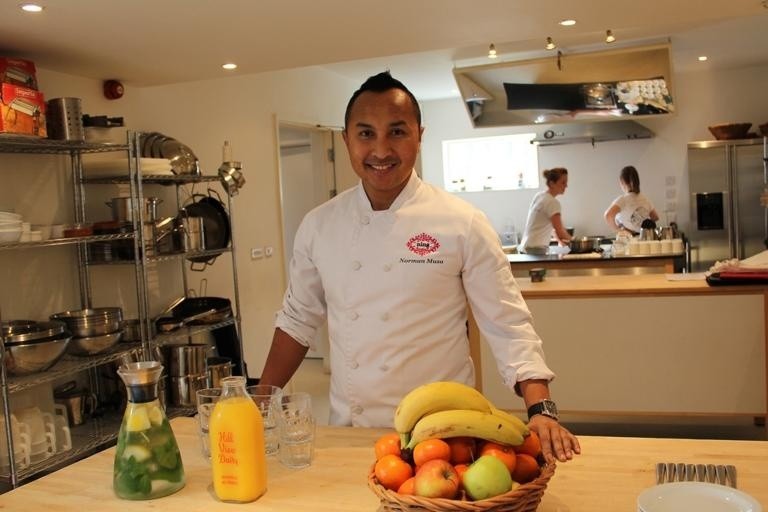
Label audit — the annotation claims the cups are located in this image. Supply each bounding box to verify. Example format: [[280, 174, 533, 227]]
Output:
[[610, 230, 628, 257], [528, 267, 546, 282], [188, 381, 314, 469], [628, 240, 682, 255]]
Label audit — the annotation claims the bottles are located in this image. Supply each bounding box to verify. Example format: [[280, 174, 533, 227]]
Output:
[[110, 356, 268, 503]]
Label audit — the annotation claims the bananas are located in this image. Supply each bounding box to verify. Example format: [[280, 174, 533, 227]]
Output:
[[392, 380, 532, 464]]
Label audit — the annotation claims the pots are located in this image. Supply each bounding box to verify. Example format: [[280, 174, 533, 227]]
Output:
[[90, 188, 234, 265], [168, 344, 237, 410], [119, 278, 235, 346]]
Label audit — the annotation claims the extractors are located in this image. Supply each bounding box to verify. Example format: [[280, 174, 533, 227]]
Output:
[[529, 118, 654, 146]]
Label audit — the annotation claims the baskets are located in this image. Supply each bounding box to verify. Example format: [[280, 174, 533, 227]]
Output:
[[368, 460, 556, 512]]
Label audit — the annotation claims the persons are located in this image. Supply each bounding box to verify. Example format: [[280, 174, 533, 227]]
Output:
[[602, 164, 660, 239], [517, 168, 573, 255], [247, 66, 584, 465]]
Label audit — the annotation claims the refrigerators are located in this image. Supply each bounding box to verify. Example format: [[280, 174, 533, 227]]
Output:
[[677, 136, 768, 272]]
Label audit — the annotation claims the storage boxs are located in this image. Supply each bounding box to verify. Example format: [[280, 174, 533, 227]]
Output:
[[0, 58, 48, 135]]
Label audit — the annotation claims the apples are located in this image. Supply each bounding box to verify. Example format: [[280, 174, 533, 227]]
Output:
[[373, 430, 543, 502]]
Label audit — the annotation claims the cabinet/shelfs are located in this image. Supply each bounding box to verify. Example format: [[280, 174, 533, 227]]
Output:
[[686, 137, 767, 275]]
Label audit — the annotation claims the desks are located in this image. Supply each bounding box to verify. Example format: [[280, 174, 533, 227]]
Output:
[[0, 415, 767, 512]]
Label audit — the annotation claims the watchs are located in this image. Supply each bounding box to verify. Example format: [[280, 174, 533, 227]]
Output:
[[527, 397, 560, 422]]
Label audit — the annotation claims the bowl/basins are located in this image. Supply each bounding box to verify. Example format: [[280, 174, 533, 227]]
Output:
[[0, 211, 67, 247], [707, 121, 753, 141], [568, 237, 601, 252], [1, 304, 126, 375], [134, 132, 201, 178]]
